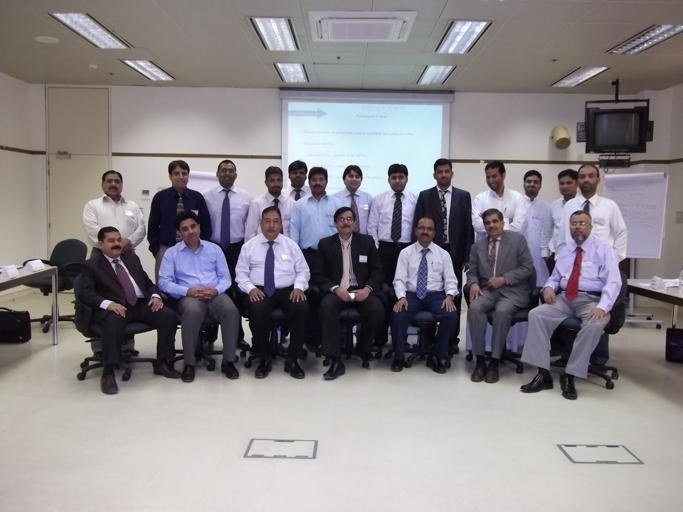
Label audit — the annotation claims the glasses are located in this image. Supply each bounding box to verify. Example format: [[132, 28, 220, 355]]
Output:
[[339, 217, 353, 224]]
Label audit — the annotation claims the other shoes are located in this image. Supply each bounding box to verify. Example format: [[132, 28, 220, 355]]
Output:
[[237, 340, 250, 350], [132, 350, 139, 356]]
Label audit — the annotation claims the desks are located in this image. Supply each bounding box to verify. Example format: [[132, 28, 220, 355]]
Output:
[[627, 278, 683, 327], [0, 266, 58, 345]]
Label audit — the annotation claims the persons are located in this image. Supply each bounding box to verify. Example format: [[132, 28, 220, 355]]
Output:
[[203, 159, 251, 351], [367, 164, 417, 347], [550, 164, 629, 366], [471, 162, 526, 244], [412, 158, 476, 354], [82, 169, 146, 360], [147, 160, 213, 283], [244, 166, 299, 245], [76, 227, 183, 394], [284, 162, 310, 201], [157, 211, 240, 382], [333, 166, 373, 236], [467, 209, 536, 383], [523, 171, 551, 289], [290, 168, 341, 331], [541, 169, 581, 277], [313, 207, 385, 381], [235, 206, 311, 380], [391, 216, 459, 373], [521, 210, 622, 400]]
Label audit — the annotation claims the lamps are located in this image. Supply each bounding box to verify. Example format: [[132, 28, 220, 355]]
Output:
[[273, 61, 309, 84], [46, 11, 131, 50], [415, 65, 458, 88], [551, 67, 610, 88], [247, 17, 299, 52], [604, 23, 683, 55], [433, 16, 493, 53], [120, 59, 176, 83]]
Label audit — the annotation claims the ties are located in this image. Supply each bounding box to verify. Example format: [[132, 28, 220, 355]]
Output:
[[273, 198, 280, 207], [176, 191, 187, 243], [264, 241, 275, 298], [112, 259, 139, 307], [340, 240, 350, 293], [293, 188, 302, 201], [440, 189, 448, 244], [391, 191, 403, 242], [488, 239, 498, 281], [416, 248, 430, 300], [583, 200, 590, 213], [349, 194, 361, 234], [567, 246, 583, 301], [220, 189, 231, 251]]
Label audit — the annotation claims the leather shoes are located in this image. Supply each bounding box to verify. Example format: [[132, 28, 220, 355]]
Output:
[[520, 371, 553, 393], [388, 349, 499, 383], [182, 364, 196, 383], [284, 359, 306, 380], [152, 358, 182, 379], [590, 360, 610, 373], [322, 361, 347, 380], [560, 373, 577, 399], [221, 359, 239, 379], [100, 367, 120, 395], [254, 361, 273, 378]]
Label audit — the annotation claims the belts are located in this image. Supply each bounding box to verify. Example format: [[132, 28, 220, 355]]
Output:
[[561, 289, 601, 296]]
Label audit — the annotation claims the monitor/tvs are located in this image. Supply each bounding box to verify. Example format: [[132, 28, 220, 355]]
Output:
[[588, 107, 644, 150]]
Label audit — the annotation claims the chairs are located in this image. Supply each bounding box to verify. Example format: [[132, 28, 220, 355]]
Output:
[[23, 239, 87, 333], [74, 279, 158, 381], [541, 258, 627, 391], [388, 248, 455, 368], [158, 288, 238, 371], [231, 286, 307, 371], [309, 283, 389, 366], [464, 283, 543, 374]]
